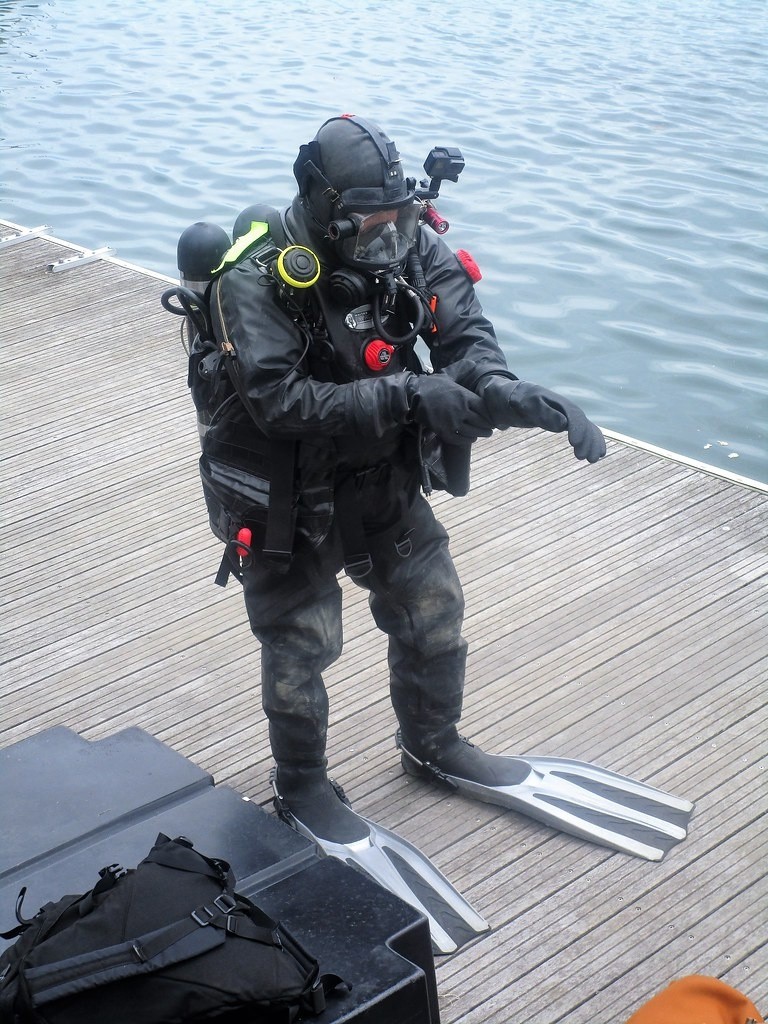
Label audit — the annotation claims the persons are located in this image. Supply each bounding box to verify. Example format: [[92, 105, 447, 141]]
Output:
[[188, 116, 691, 953]]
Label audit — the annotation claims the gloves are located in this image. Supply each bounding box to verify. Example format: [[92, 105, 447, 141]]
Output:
[[474, 373, 606, 464], [406, 359, 495, 447]]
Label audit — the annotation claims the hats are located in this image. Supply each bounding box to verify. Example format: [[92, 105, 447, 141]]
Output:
[[291, 116, 421, 277]]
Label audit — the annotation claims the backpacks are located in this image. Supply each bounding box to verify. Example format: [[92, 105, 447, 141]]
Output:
[[0, 831, 353, 1024]]
